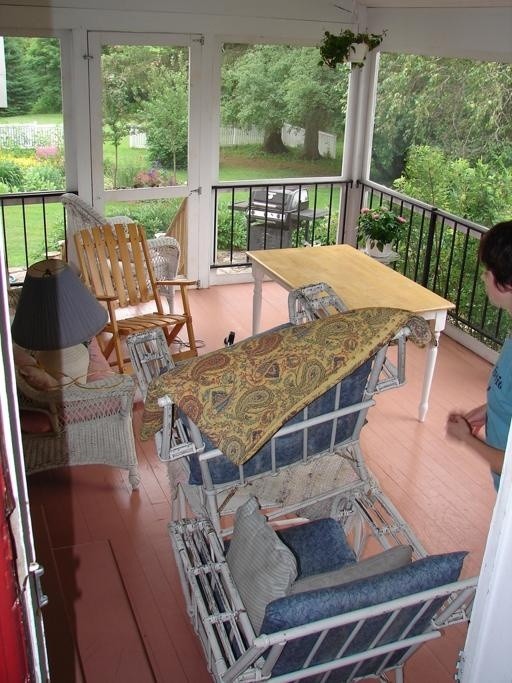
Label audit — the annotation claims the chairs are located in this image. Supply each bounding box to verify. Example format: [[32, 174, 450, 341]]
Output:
[[60, 193, 182, 313], [168, 473, 480, 683], [125, 283, 412, 551], [74, 221, 199, 376]]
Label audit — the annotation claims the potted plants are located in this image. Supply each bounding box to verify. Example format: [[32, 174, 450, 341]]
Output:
[[317, 25, 388, 73], [358, 207, 406, 259]]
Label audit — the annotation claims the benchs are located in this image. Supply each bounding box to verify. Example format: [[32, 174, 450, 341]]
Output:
[[9, 286, 141, 494]]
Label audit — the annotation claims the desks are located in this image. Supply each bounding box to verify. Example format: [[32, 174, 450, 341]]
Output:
[[245, 243, 456, 424]]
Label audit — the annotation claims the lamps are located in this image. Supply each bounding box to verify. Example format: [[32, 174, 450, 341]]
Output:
[[12, 258, 110, 387]]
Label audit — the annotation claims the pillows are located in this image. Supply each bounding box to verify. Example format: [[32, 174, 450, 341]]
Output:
[[220, 496, 298, 640], [285, 545, 413, 598]]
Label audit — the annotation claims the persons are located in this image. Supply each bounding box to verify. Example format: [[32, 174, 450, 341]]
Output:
[[445, 220, 512, 492]]
